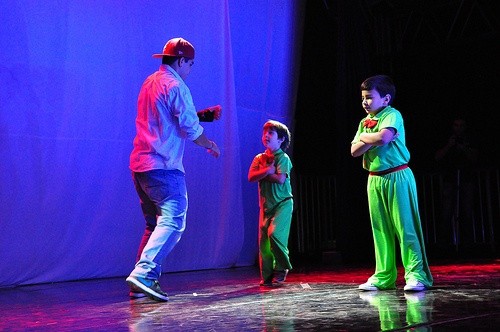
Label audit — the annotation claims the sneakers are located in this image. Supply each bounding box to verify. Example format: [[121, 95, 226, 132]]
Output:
[[359, 281, 378, 290], [129, 290, 146, 298], [126, 276, 169, 302], [404, 281, 425, 291]]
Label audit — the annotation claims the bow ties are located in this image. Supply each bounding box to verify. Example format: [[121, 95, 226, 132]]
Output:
[[262, 148, 274, 159], [363, 119, 378, 128]]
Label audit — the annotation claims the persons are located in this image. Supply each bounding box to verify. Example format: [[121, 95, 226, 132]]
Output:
[[350, 76, 434, 291], [125, 37, 222, 302], [247, 120, 294, 286]]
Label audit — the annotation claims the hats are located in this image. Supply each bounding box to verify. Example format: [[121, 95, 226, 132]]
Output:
[[152, 38, 195, 58]]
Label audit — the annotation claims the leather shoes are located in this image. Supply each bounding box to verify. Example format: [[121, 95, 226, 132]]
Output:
[[274, 269, 289, 282]]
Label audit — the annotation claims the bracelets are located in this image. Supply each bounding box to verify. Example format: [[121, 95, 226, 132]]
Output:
[[207, 141, 215, 149]]
